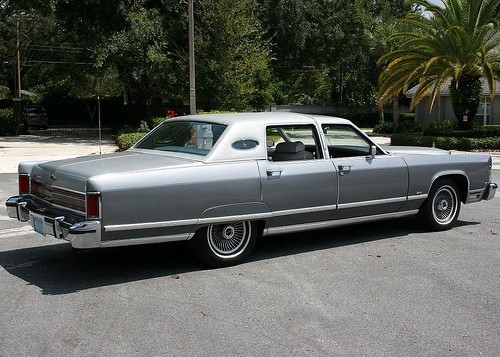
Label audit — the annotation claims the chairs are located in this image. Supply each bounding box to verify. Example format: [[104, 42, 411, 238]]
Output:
[[272, 141, 314, 160]]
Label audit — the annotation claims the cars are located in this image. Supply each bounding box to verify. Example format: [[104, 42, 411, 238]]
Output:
[[5, 112, 497, 269]]
